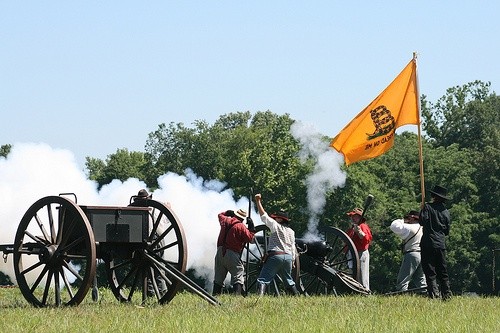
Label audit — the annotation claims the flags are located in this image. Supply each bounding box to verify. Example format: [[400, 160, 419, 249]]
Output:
[[329, 60, 418, 166]]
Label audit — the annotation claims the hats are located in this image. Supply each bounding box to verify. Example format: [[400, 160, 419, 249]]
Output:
[[346, 208, 366, 220], [405, 210, 419, 219], [234, 209, 246, 220], [271, 210, 289, 221], [426, 185, 451, 201], [133, 189, 152, 201]]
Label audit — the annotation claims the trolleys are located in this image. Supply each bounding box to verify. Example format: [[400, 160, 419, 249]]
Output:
[[0, 188, 223, 308], [230, 216, 440, 297]]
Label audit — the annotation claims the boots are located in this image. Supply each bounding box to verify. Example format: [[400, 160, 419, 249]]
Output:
[[287, 284, 300, 297], [233, 283, 242, 296], [211, 282, 222, 296], [257, 283, 268, 296]]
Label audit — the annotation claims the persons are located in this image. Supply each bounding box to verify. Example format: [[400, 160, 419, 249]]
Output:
[[134, 190, 171, 296], [255, 194, 300, 296], [418, 185, 452, 303], [344, 207, 373, 290], [212, 208, 255, 296], [390, 210, 427, 296]]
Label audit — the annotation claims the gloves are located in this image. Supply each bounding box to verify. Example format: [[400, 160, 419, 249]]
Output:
[[353, 224, 365, 239]]
